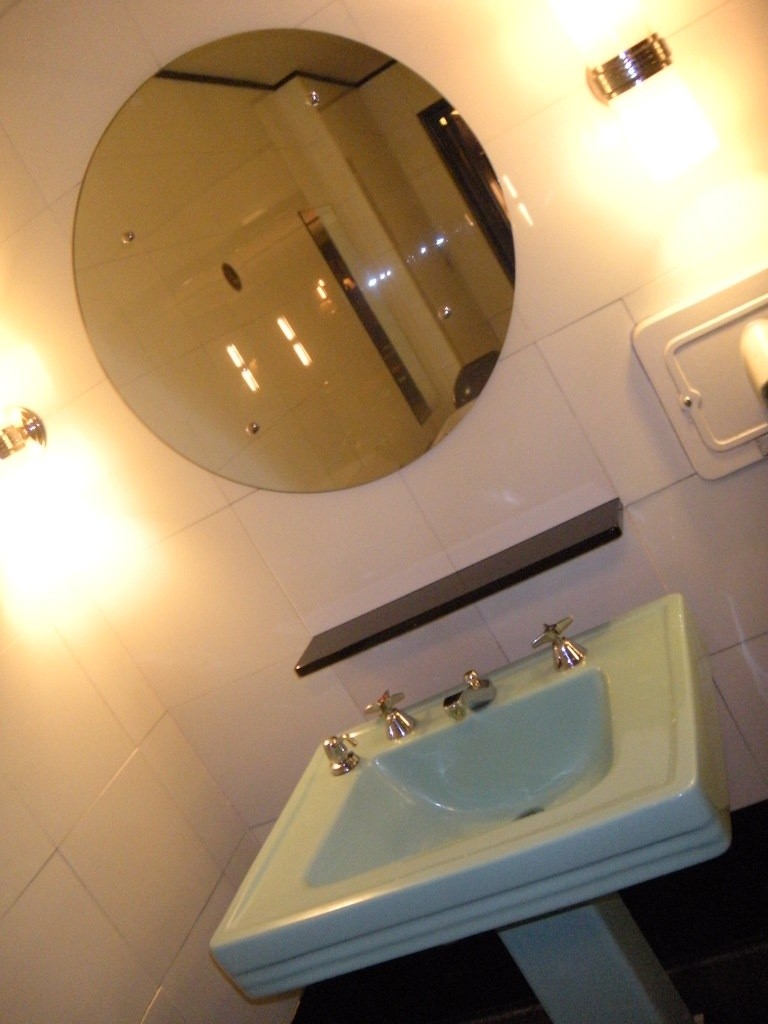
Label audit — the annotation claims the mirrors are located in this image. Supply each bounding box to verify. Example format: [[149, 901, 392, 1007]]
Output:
[[70, 27, 517, 494]]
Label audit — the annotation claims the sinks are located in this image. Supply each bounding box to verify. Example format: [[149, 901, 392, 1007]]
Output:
[[210, 593, 732, 1000]]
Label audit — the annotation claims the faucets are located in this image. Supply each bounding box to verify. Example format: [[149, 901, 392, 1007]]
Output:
[[443, 671, 495, 721]]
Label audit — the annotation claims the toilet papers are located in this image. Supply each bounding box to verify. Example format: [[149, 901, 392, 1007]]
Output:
[[739, 319, 767, 407]]
